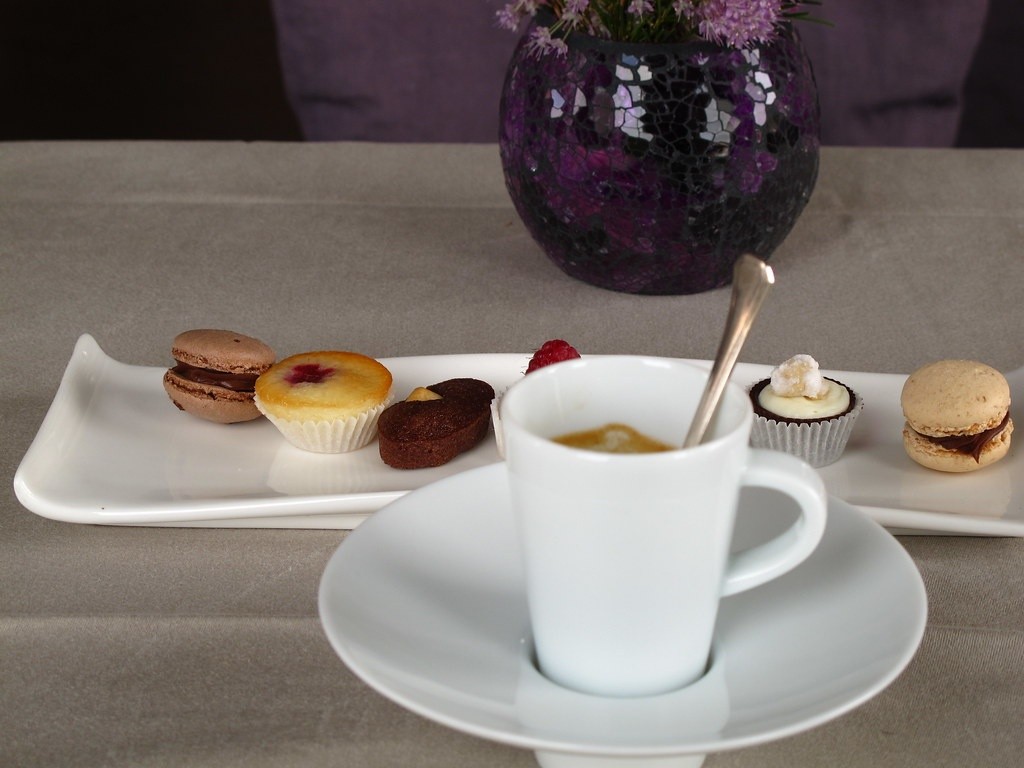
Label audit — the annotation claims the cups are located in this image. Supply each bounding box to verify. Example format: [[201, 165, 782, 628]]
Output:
[[494, 349, 828, 697]]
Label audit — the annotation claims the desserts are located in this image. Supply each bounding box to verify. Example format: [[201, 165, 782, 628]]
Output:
[[746, 353, 864, 470], [903, 358, 1015, 472], [162, 329, 275, 424], [254, 350, 394, 455]]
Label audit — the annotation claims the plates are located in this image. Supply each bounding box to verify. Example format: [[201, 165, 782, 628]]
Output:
[[11, 334, 1024, 546]]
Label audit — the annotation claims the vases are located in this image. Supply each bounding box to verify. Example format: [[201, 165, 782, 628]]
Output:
[[499, 20, 822, 296]]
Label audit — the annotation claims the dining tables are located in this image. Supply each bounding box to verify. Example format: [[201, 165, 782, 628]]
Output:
[[0, 140, 1024, 768]]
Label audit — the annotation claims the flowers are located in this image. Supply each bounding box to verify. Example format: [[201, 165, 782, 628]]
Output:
[[495, 0, 836, 65]]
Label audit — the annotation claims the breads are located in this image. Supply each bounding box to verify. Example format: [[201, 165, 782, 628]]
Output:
[[378, 377, 495, 469]]
